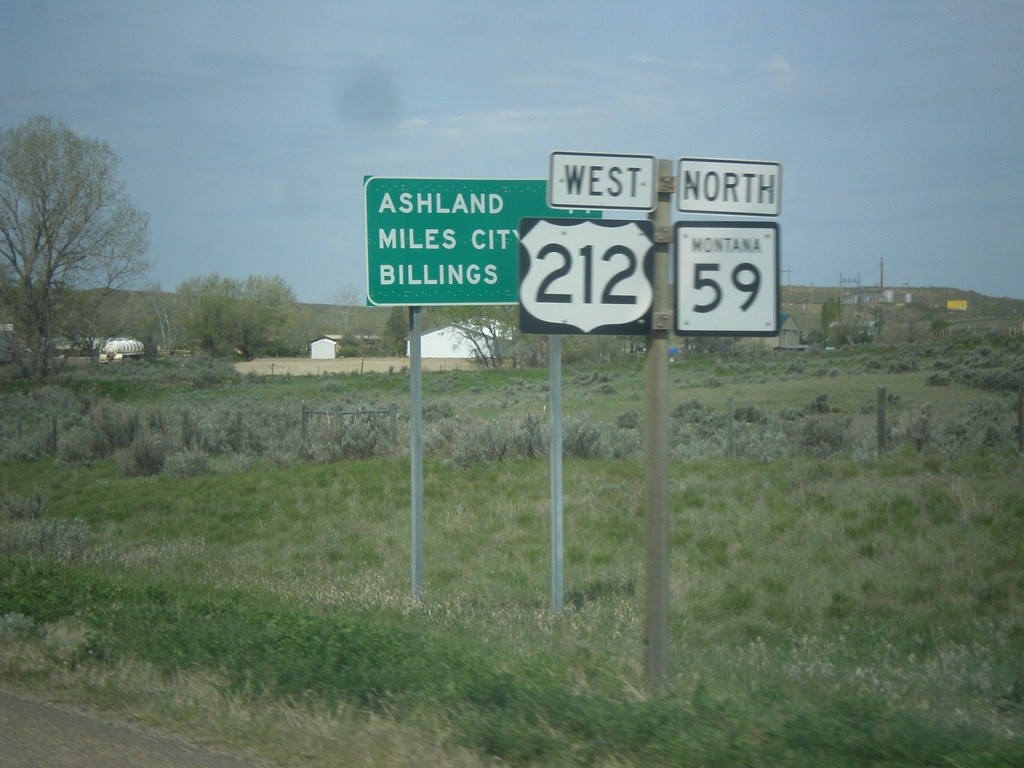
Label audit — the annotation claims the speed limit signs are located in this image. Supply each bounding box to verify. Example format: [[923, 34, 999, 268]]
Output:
[[672, 220, 781, 338]]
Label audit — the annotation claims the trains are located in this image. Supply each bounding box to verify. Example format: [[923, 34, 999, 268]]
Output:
[[98, 339, 144, 362]]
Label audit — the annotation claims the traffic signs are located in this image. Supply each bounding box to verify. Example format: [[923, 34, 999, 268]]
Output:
[[362, 176, 605, 307]]
